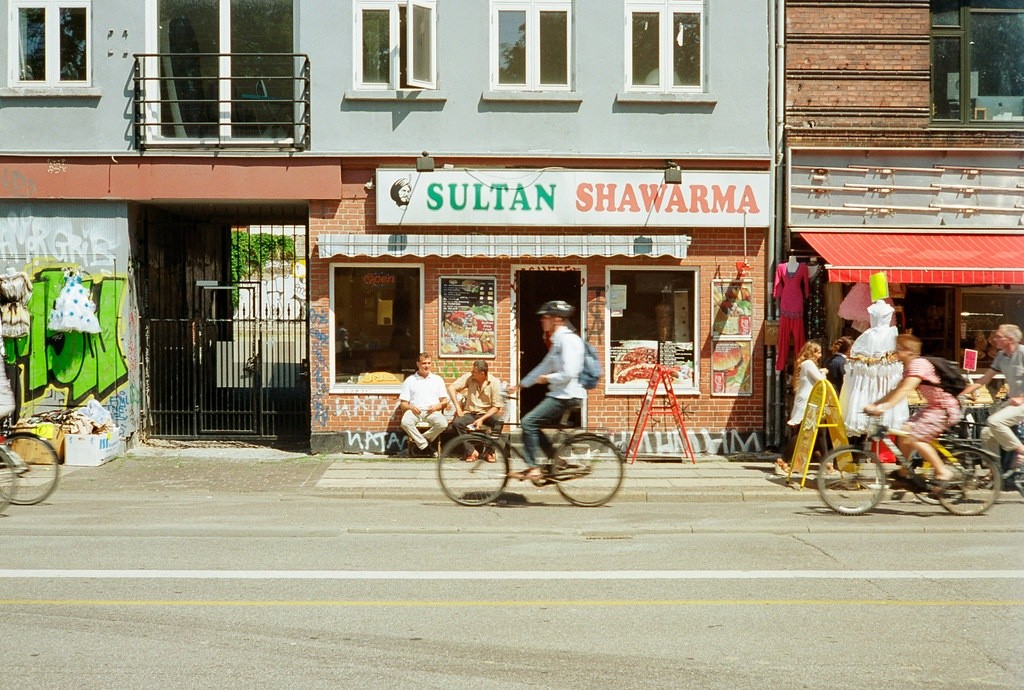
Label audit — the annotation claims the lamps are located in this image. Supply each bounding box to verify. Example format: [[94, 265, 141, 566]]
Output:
[[664, 159, 681, 184], [416, 150, 434, 172]]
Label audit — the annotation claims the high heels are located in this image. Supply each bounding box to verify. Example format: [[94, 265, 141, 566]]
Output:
[[775, 459, 792, 476], [819, 461, 841, 476]]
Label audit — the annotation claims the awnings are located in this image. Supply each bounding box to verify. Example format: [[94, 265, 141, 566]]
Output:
[[316, 235, 692, 260], [798, 232, 1024, 283]]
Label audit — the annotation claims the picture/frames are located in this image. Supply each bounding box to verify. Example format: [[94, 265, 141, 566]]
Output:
[[439, 276, 497, 358], [711, 338, 753, 397], [711, 279, 753, 338]]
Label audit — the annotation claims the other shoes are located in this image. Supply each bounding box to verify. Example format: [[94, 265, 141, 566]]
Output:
[[423, 439, 439, 458], [506, 467, 543, 479], [482, 453, 496, 462], [411, 443, 420, 456], [465, 455, 478, 462]]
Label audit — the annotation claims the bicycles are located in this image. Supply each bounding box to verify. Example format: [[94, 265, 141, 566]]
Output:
[[909, 404, 1024, 505], [0, 412, 61, 514], [437, 382, 626, 507], [816, 409, 1002, 515]]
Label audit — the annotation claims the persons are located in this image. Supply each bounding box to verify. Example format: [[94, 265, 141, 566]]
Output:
[[865, 335, 959, 497], [959, 323, 1024, 484], [775, 337, 856, 475], [447, 360, 504, 462], [399, 353, 450, 458], [506, 300, 586, 480], [773, 254, 846, 369], [843, 300, 910, 437], [0, 266, 34, 339]]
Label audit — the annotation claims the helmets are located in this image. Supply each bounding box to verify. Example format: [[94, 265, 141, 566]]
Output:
[[536, 300, 574, 318]]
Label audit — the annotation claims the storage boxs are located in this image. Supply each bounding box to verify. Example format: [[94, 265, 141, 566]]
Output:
[[7, 418, 67, 466], [61, 426, 121, 468]]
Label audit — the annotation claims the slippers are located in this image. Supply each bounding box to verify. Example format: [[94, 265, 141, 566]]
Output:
[[890, 471, 909, 480], [927, 472, 959, 494]]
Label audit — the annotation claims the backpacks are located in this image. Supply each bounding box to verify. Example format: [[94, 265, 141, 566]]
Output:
[[579, 339, 602, 390], [927, 356, 967, 396]]
